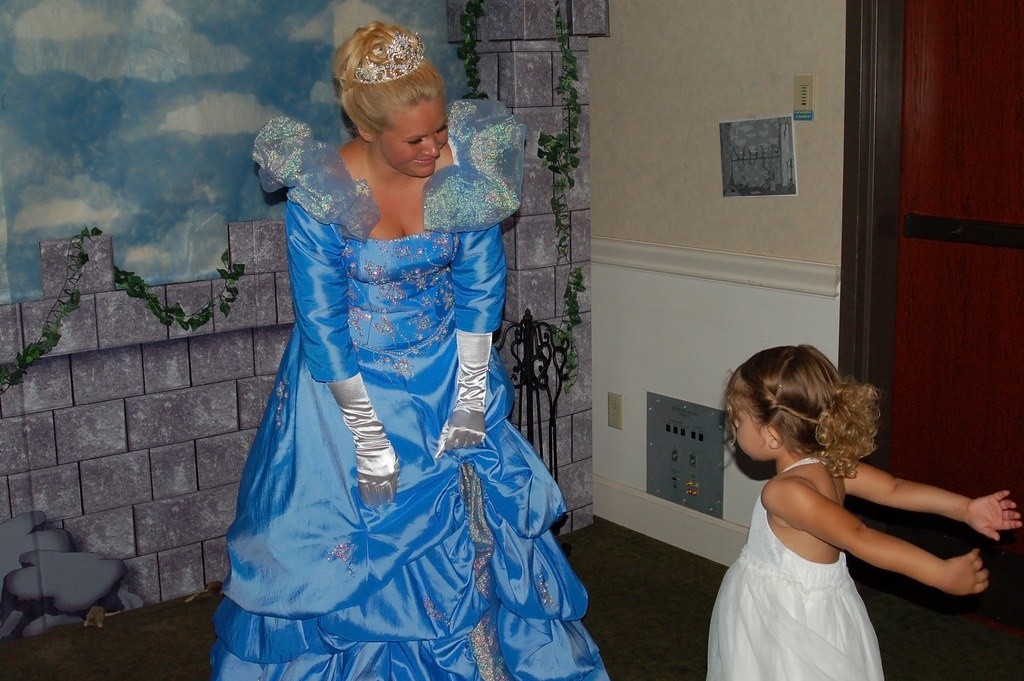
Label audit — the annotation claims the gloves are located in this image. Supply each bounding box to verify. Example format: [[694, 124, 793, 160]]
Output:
[[329, 373, 400, 506], [436, 328, 493, 458]]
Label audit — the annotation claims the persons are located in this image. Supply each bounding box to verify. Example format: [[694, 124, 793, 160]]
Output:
[[705, 345, 1022, 681], [208, 21, 611, 681]]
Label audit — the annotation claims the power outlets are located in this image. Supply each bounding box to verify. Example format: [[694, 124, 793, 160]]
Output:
[[606, 392, 621, 428]]
